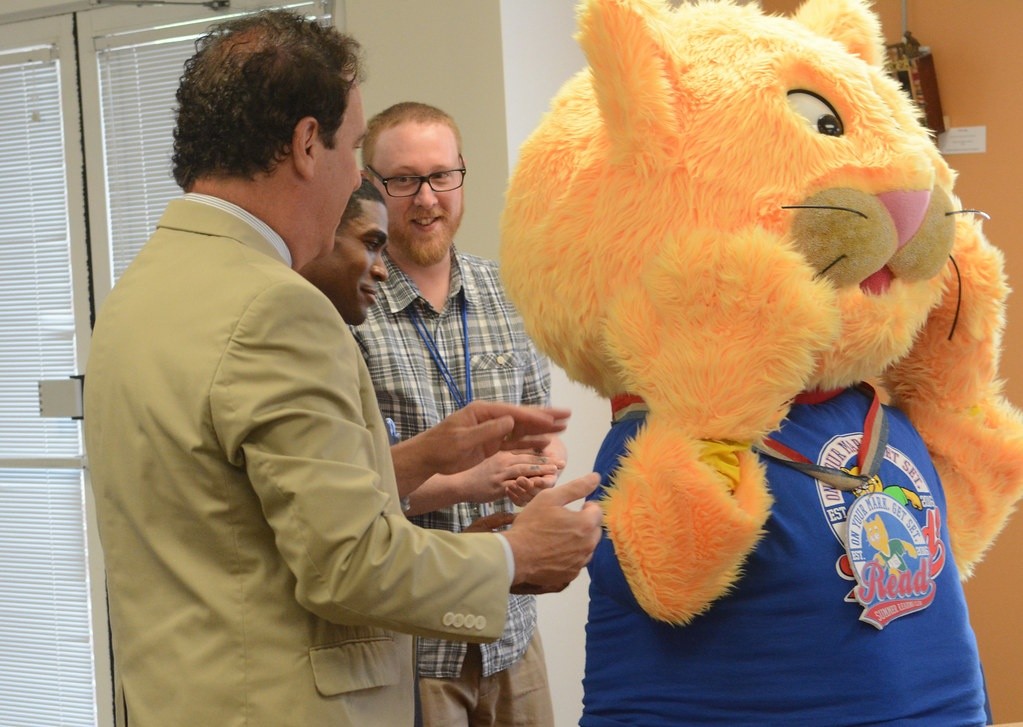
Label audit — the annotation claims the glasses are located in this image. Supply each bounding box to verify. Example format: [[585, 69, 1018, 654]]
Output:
[[366, 154, 466, 197]]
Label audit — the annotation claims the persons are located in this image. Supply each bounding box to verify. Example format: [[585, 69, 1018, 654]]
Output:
[[83, 11, 603, 727], [350, 102, 568, 727]]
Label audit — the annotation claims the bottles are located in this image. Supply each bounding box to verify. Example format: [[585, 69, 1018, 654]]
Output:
[[381, 418, 410, 514]]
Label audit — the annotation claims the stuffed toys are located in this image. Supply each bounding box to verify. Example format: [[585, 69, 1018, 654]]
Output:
[[503, 0, 1023, 727]]
